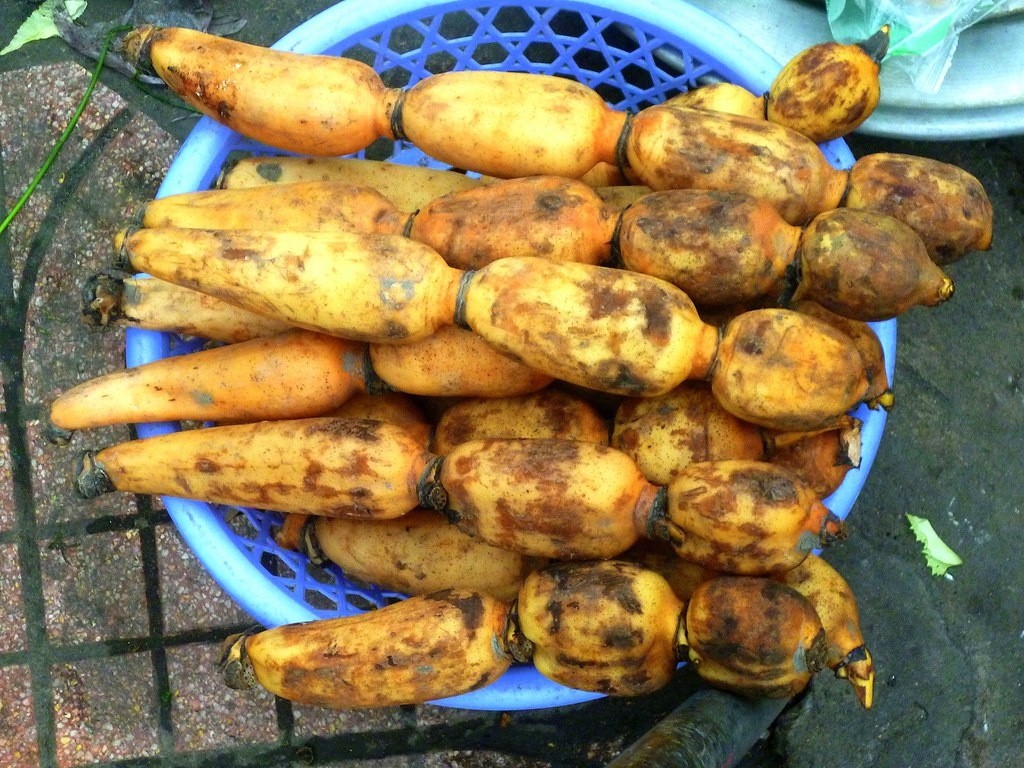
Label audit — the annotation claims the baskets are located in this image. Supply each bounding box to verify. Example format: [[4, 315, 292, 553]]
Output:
[[122, 0, 898, 712]]
